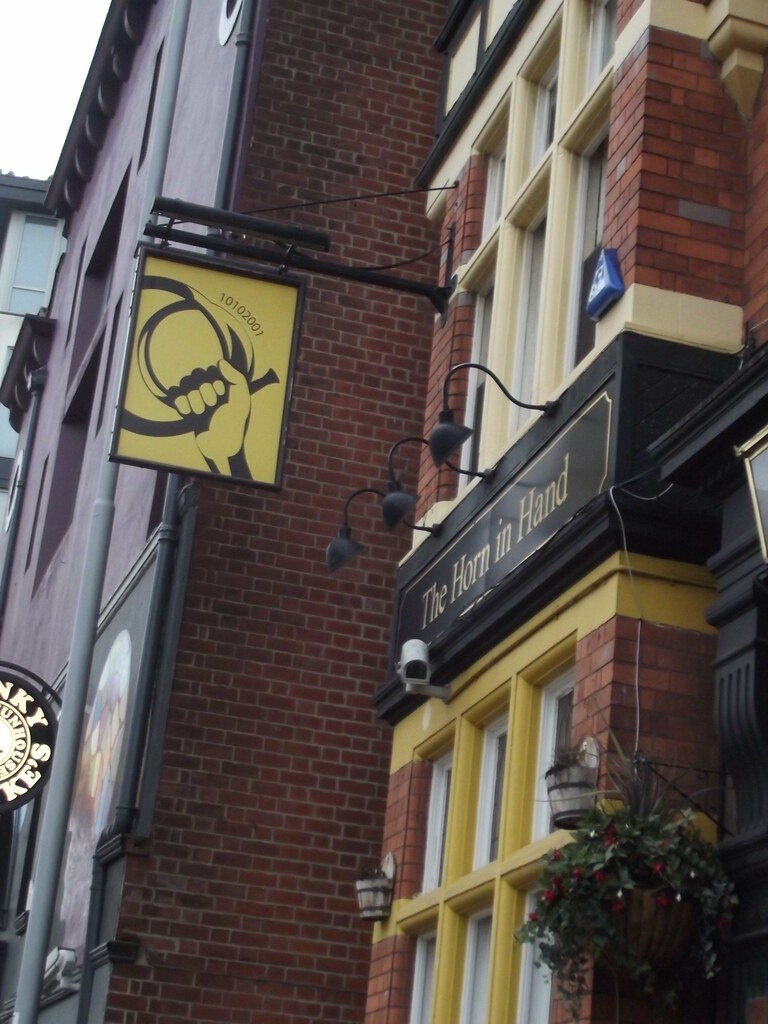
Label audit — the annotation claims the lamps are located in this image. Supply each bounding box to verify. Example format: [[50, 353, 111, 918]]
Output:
[[545, 751, 596, 829], [326, 488, 444, 573], [354, 865, 392, 921], [429, 363, 559, 466], [382, 437, 496, 532]]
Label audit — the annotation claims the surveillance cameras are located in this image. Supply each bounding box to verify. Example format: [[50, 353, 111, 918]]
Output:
[[43, 946, 77, 995], [400, 638, 432, 684]]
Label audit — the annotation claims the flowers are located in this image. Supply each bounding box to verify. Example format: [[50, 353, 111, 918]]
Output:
[[513, 755, 741, 1024]]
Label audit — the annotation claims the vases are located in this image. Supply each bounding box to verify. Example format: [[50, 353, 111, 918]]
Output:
[[608, 888, 701, 970]]
